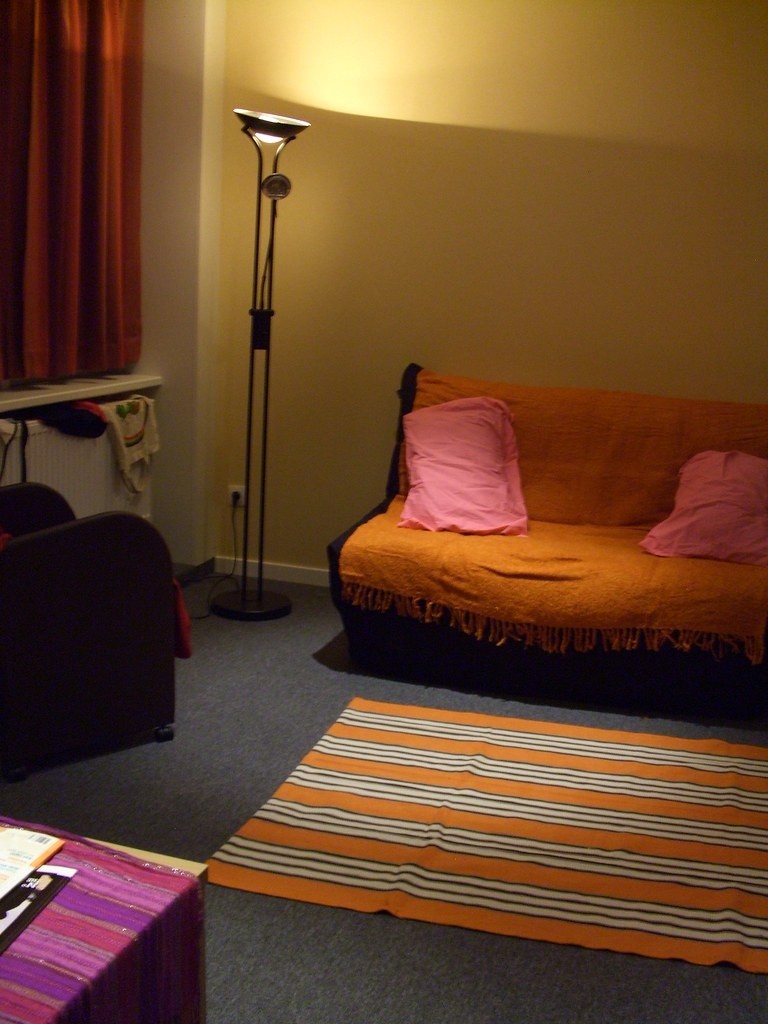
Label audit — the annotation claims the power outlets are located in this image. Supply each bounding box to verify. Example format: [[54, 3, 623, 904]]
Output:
[[227, 485, 247, 508]]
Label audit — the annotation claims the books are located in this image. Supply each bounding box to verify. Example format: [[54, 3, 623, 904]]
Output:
[[0, 827, 79, 957]]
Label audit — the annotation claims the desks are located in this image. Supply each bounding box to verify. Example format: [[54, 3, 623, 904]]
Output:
[[0, 814, 211, 1024]]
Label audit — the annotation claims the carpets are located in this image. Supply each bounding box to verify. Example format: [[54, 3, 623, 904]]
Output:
[[203, 697, 768, 977]]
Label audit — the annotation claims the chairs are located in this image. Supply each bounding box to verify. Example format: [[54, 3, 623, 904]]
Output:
[[0, 485, 176, 786]]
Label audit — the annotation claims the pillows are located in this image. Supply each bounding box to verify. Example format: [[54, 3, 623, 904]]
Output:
[[395, 398, 529, 537], [646, 449, 768, 566]]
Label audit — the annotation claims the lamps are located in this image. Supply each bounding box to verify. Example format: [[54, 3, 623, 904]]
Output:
[[212, 107, 313, 622]]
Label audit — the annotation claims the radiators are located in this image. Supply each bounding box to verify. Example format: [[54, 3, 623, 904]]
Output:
[[0, 395, 160, 540]]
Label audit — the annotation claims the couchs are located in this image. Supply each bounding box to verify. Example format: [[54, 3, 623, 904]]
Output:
[[326, 363, 768, 735]]
